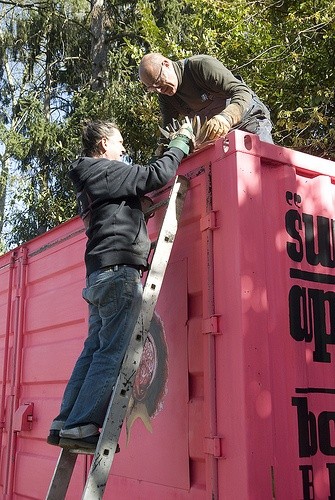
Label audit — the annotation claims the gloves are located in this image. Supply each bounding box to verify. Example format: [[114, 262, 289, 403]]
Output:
[[168, 123, 194, 158], [202, 103, 243, 141]]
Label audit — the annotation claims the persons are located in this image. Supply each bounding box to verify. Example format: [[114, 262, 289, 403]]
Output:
[[139, 53, 275, 157], [45, 118, 196, 453]]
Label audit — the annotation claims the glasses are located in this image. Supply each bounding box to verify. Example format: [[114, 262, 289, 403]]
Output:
[[142, 63, 164, 92]]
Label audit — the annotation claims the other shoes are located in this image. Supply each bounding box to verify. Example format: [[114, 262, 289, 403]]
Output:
[[59, 431, 120, 453], [46, 429, 60, 447]]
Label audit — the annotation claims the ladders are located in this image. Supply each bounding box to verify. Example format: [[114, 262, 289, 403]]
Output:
[[44, 174, 191, 500]]
